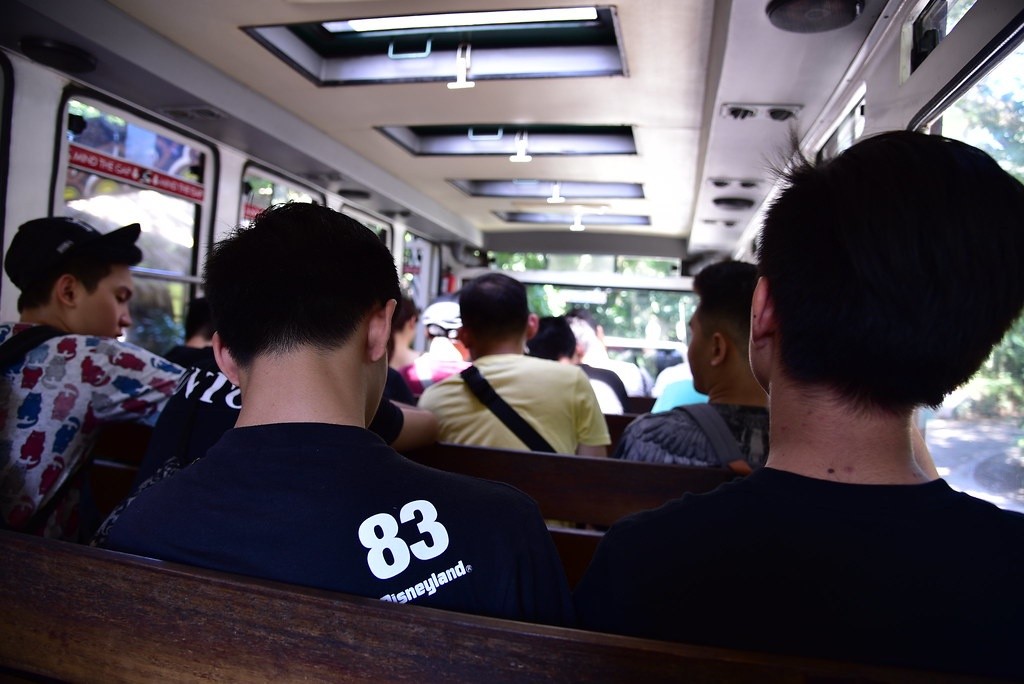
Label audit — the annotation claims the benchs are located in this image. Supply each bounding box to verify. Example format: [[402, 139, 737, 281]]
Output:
[[0, 341, 1024, 684]]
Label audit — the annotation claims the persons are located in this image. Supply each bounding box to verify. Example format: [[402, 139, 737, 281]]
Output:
[[1, 122, 1023, 674]]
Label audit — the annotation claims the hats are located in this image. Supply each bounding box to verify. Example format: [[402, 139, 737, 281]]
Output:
[[4, 217, 142, 292]]
